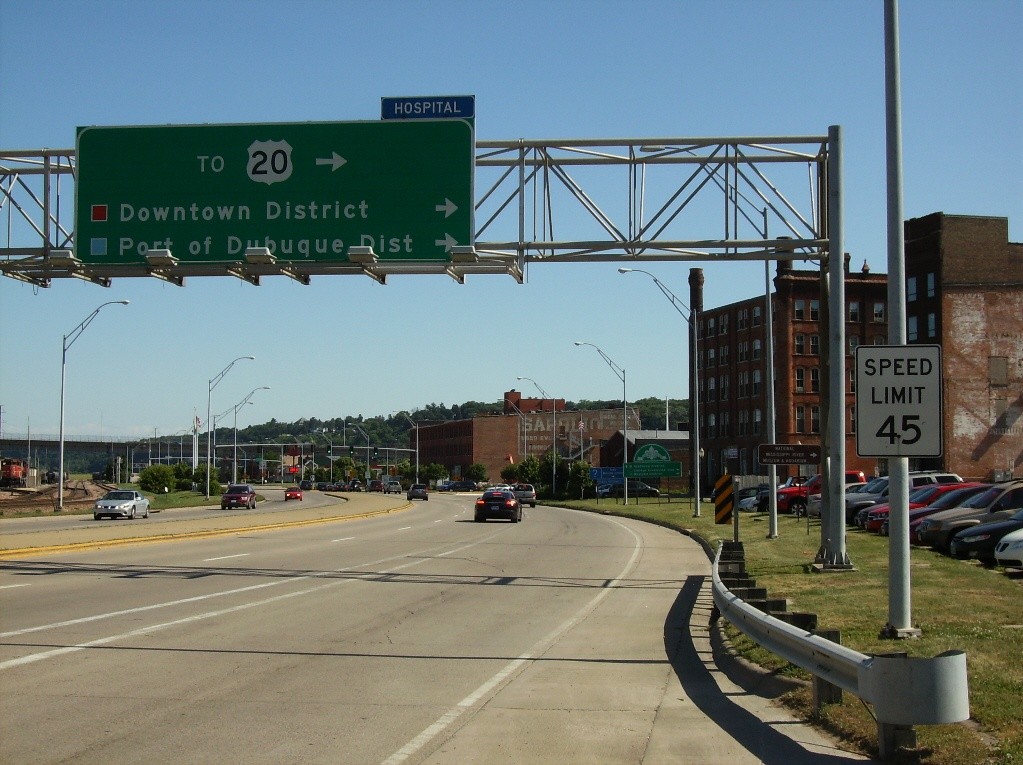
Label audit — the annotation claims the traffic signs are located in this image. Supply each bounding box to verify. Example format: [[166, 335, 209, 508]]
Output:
[[758, 444, 821, 466], [74, 117, 478, 267]]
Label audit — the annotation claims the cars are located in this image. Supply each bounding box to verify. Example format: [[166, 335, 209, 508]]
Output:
[[283, 487, 302, 501], [486, 482, 515, 492], [474, 491, 522, 524], [94, 489, 150, 519], [438, 481, 476, 492]]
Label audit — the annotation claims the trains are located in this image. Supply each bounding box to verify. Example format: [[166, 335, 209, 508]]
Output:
[[0, 457, 28, 488]]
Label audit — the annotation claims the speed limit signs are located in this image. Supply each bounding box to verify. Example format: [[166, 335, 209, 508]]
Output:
[[855, 346, 943, 458]]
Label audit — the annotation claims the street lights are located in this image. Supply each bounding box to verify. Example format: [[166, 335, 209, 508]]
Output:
[[58, 299, 270, 512], [497, 267, 704, 518]]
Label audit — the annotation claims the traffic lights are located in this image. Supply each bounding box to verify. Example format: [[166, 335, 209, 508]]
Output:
[[328, 445, 379, 456]]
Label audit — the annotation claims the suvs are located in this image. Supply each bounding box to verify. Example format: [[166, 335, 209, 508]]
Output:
[[757, 467, 1022, 574], [301, 480, 428, 501]]
